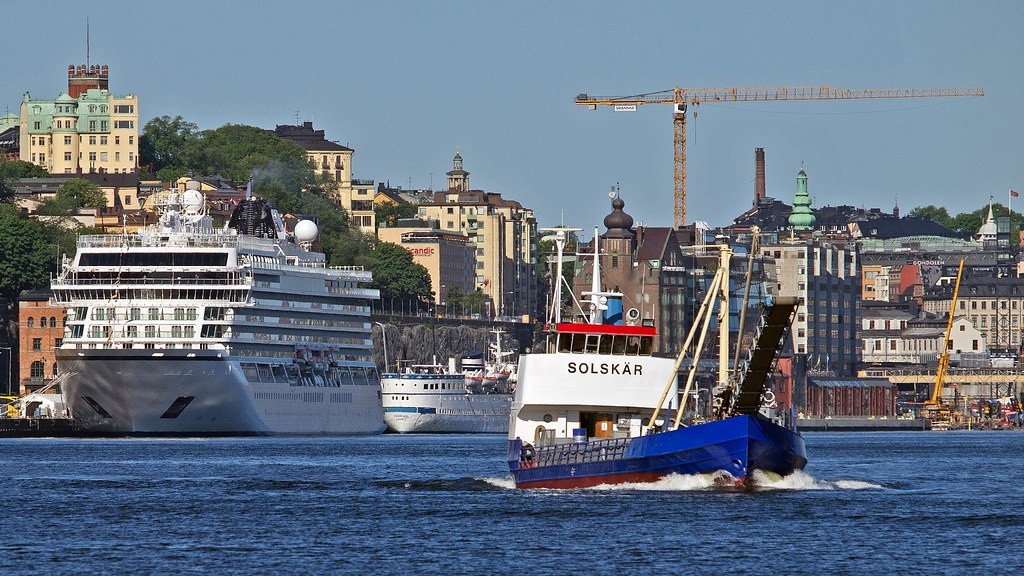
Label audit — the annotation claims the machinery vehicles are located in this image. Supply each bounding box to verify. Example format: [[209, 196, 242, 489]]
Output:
[[919, 256, 966, 430]]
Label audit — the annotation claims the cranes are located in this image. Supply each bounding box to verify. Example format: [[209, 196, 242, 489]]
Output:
[[571, 85, 986, 233]]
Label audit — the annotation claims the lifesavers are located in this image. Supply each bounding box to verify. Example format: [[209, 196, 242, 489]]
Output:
[[521, 443, 536, 467]]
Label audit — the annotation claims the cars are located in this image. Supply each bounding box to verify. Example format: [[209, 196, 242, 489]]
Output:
[[499, 315, 522, 324], [894, 395, 1024, 429], [0, 394, 58, 429]]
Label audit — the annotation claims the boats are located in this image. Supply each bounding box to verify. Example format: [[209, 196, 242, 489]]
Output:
[[507, 226, 809, 492], [50, 174, 390, 438], [382, 325, 516, 434]]
